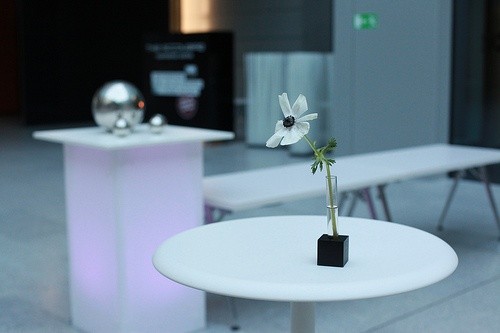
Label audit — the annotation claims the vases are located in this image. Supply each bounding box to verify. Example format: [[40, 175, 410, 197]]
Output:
[[326, 176, 340, 238]]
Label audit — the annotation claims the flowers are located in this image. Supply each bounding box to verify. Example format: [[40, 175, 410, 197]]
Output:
[[267, 92, 339, 237]]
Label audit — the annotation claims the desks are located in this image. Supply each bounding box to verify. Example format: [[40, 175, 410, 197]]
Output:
[[31, 121, 235, 332], [152, 214, 459, 333], [203, 143, 500, 233]]
